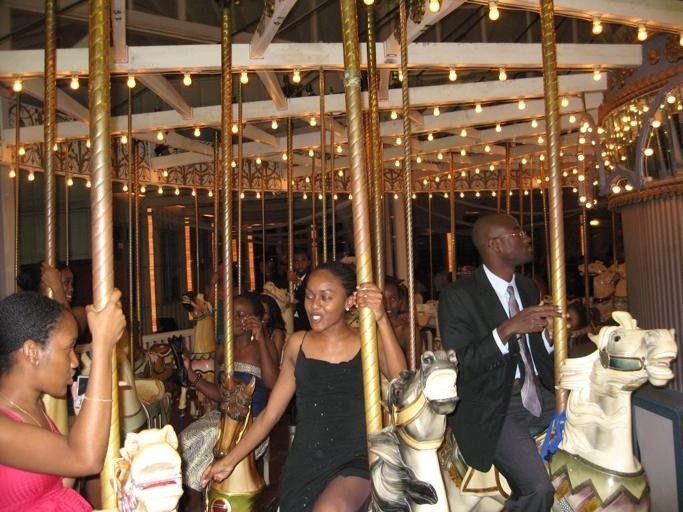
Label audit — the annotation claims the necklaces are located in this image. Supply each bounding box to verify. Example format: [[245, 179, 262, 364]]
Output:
[[2, 394, 53, 431]]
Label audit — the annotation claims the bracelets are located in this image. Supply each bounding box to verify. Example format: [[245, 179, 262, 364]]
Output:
[[85, 396, 113, 402], [193, 377, 199, 387]]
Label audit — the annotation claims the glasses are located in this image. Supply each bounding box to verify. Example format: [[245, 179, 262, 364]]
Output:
[[493, 229, 527, 241]]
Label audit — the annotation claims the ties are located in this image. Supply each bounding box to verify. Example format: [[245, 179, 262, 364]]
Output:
[[506, 285, 544, 418]]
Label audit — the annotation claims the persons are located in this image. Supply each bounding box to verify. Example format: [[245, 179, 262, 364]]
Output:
[[201, 264, 407, 512], [382, 267, 475, 367], [438, 214, 571, 512], [42, 262, 92, 347], [0, 288, 126, 512], [173, 250, 358, 468], [566, 303, 583, 357]]
